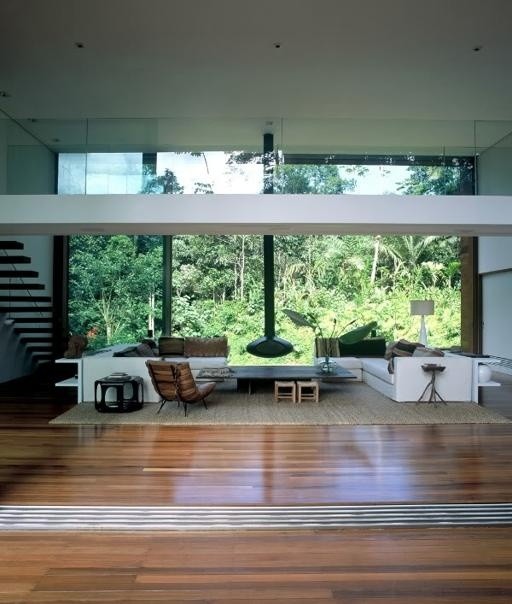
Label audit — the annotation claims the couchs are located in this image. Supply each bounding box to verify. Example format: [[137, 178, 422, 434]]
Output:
[[82, 334, 231, 404], [312, 333, 475, 405]]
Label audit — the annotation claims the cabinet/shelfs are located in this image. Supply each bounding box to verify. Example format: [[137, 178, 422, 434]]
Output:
[[443, 349, 501, 405], [51, 341, 119, 406]]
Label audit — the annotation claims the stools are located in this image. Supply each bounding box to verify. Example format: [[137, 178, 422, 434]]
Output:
[[273, 379, 297, 403], [298, 380, 321, 404], [416, 361, 448, 409]]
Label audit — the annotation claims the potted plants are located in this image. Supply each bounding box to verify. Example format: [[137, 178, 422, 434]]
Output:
[[281, 307, 379, 374]]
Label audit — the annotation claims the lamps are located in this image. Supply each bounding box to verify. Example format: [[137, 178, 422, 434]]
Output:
[[407, 298, 436, 348]]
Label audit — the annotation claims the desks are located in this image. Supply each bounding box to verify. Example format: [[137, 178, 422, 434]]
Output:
[[92, 374, 146, 415]]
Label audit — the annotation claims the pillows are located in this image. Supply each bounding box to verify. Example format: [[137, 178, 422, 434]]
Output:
[[158, 334, 183, 358], [314, 334, 339, 358], [339, 338, 358, 356], [111, 345, 140, 362], [384, 339, 400, 359], [387, 355, 395, 374], [184, 336, 229, 358], [390, 337, 425, 357], [410, 345, 444, 357], [355, 339, 385, 360], [134, 342, 156, 357]]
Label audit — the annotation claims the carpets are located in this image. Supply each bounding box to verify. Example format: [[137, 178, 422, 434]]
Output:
[[48, 373, 512, 428]]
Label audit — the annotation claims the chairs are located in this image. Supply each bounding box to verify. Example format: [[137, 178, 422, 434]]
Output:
[[142, 358, 219, 419]]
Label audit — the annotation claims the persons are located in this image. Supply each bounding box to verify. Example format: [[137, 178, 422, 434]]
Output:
[[86, 326, 98, 336]]
